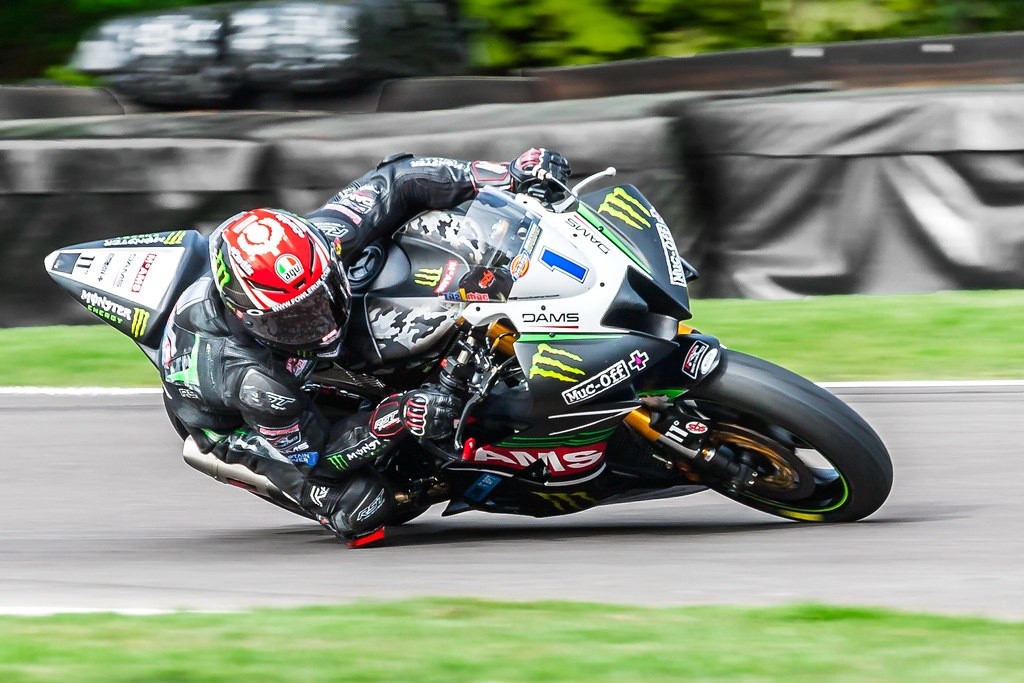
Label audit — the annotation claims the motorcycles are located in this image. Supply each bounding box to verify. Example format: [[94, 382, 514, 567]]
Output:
[[47, 158, 895, 525]]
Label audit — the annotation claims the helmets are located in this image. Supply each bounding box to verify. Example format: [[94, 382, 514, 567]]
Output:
[[208, 208, 352, 359]]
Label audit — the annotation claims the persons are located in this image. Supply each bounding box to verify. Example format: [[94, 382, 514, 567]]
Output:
[[156, 146, 573, 537]]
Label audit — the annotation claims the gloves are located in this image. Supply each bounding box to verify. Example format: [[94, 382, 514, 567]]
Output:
[[473, 147, 572, 201], [371, 383, 462, 440]]
